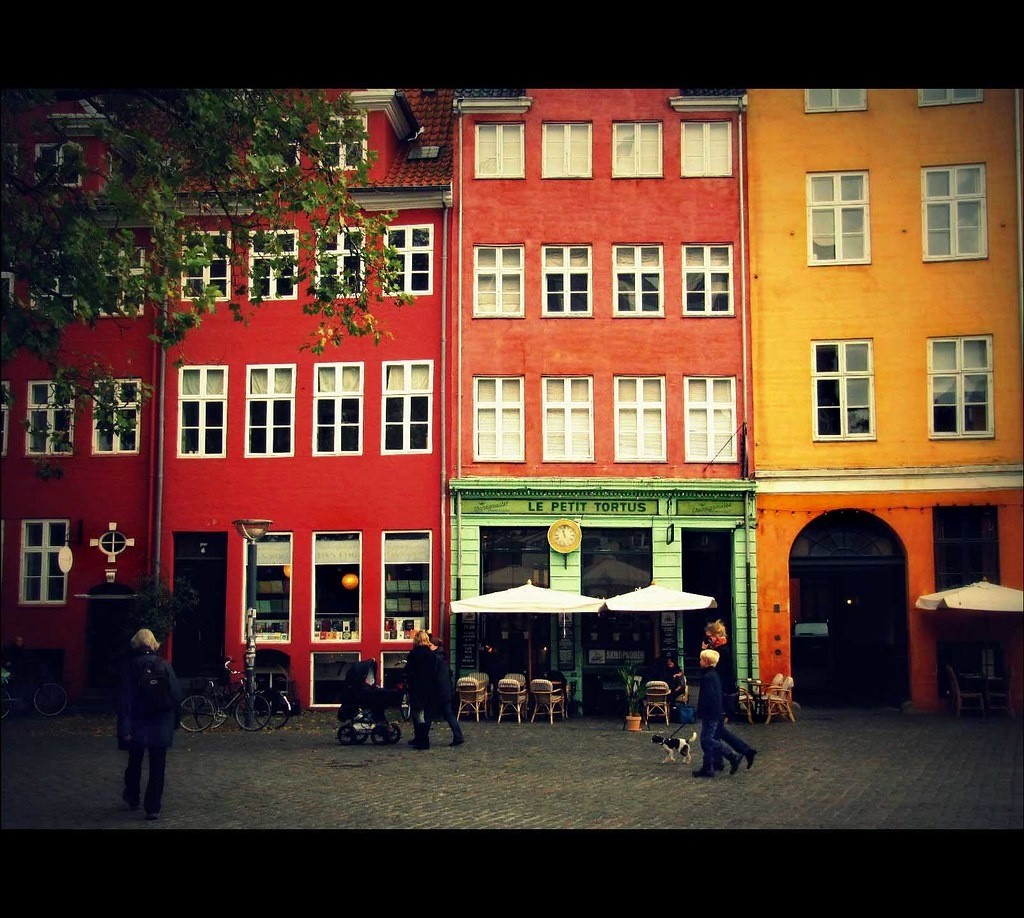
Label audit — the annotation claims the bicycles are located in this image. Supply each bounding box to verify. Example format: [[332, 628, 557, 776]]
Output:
[[0, 668, 69, 719], [177, 658, 293, 733], [394, 660, 411, 721]]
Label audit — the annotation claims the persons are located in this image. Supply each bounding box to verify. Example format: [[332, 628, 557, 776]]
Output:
[[691, 650, 743, 777], [701, 622, 757, 772], [641, 657, 685, 709], [116, 629, 184, 819], [0, 636, 30, 714], [404, 630, 465, 746]]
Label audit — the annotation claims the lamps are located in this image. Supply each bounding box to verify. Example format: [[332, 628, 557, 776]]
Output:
[[338, 568, 360, 592], [281, 566, 290, 579]]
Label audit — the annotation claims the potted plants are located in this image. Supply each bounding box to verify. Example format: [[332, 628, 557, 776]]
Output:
[[615, 661, 650, 732]]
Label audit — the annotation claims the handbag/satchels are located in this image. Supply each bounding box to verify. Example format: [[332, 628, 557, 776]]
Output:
[[676, 704, 696, 724]]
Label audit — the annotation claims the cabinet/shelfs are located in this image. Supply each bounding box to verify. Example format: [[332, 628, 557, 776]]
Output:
[[255, 580, 288, 620], [385, 581, 430, 617]]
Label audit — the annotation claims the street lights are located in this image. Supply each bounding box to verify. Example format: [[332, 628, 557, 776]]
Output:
[[232, 519, 275, 730]]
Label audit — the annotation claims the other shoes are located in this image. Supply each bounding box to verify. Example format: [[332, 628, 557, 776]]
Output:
[[730, 754, 743, 774], [693, 767, 713, 777], [413, 743, 428, 749], [712, 760, 724, 771], [123, 788, 140, 810], [746, 749, 757, 769], [408, 738, 418, 745], [449, 738, 464, 746], [147, 810, 159, 818]]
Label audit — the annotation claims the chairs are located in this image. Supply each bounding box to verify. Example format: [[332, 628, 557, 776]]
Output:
[[455, 677, 488, 719], [502, 674, 526, 709], [528, 676, 567, 725], [470, 672, 493, 716], [644, 680, 671, 726], [945, 664, 985, 716], [760, 673, 785, 716], [724, 684, 755, 725], [944, 663, 974, 711], [497, 678, 528, 725], [764, 676, 797, 725], [990, 662, 1015, 721], [675, 675, 689, 704]]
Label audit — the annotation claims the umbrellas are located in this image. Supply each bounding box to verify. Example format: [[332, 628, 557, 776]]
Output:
[[483, 565, 548, 588], [584, 555, 651, 587], [915, 577, 1024, 695], [451, 579, 606, 681], [604, 584, 718, 654]]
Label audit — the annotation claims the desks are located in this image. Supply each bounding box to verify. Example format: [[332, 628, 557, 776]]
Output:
[[551, 681, 563, 721], [746, 681, 772, 718], [963, 671, 1005, 714]]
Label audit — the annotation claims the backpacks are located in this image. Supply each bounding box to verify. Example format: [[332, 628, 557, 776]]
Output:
[[133, 654, 176, 717]]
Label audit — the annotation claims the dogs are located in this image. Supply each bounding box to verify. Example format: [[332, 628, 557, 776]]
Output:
[[651, 732, 697, 765]]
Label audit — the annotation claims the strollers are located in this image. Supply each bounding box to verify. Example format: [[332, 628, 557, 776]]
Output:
[[335, 658, 402, 745]]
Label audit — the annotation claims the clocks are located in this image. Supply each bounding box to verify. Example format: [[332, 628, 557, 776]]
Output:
[[547, 518, 583, 554]]
